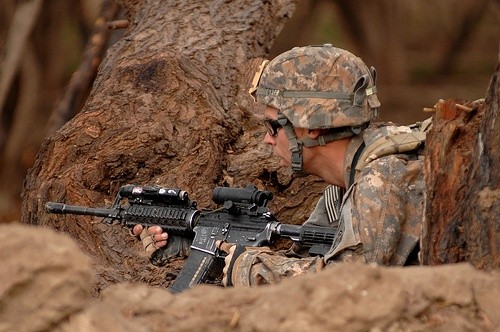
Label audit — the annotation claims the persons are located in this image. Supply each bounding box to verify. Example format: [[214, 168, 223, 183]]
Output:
[[128, 43, 432, 287]]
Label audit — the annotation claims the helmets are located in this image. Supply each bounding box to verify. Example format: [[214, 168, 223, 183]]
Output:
[[250, 43, 381, 130]]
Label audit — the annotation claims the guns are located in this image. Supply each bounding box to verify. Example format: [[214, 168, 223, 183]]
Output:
[[45, 184, 338, 293]]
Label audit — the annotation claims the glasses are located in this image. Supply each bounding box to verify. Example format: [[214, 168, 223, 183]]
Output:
[[265, 118, 289, 136]]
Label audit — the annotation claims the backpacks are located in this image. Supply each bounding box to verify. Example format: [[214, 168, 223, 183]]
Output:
[[355, 118, 435, 181]]
